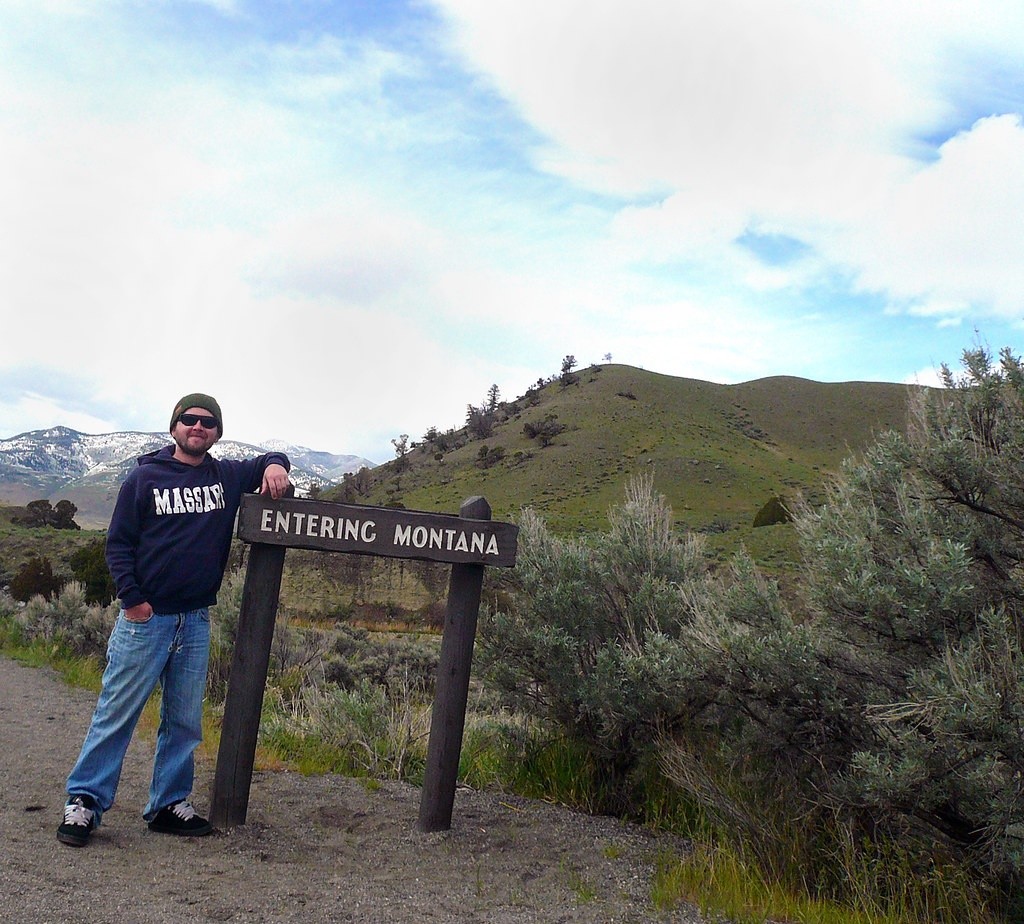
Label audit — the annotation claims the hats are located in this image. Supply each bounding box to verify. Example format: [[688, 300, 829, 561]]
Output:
[[170, 393, 223, 439]]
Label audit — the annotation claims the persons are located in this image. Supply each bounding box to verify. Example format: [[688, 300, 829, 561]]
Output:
[[56, 393, 290, 847]]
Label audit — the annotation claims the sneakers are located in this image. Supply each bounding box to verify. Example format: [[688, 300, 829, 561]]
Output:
[[57, 794, 94, 845], [148, 798, 211, 836]]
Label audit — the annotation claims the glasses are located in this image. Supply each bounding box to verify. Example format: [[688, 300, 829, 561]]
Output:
[[178, 414, 219, 429]]
